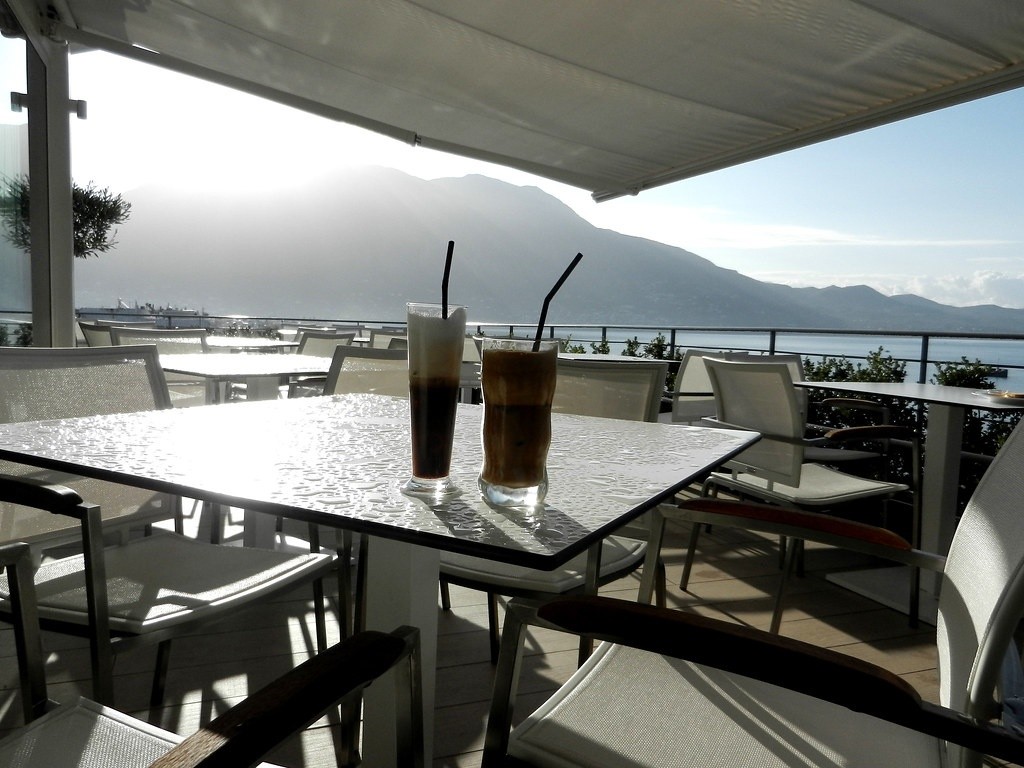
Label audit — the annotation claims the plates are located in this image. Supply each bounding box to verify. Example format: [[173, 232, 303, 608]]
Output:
[[972, 391, 1024, 403]]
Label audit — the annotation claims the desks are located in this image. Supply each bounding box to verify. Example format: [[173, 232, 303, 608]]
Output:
[[795, 380, 1024, 623], [0, 393, 759, 768], [125, 353, 333, 549], [146, 336, 300, 353], [557, 352, 681, 368]]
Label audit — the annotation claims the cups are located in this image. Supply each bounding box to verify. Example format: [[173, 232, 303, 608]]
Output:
[[398, 302, 469, 500], [477, 335, 559, 508]]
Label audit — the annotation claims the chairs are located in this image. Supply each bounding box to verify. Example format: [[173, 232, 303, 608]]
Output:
[[435, 364, 668, 670], [78, 321, 478, 395], [678, 356, 918, 635], [0, 344, 334, 728], [0, 541, 424, 768], [482, 416, 1024, 768]]
